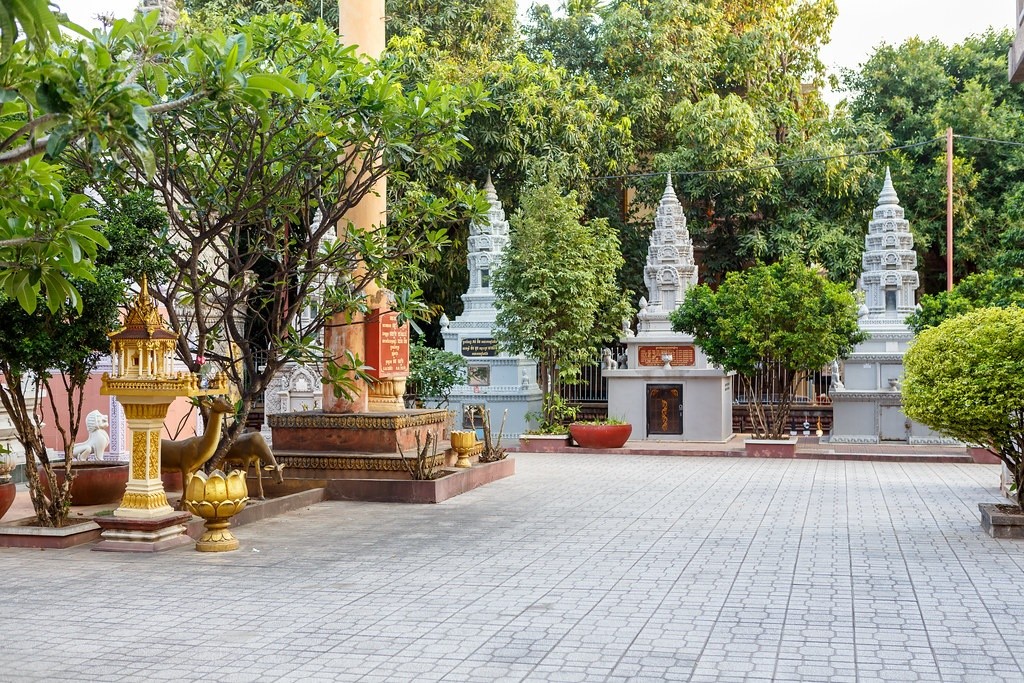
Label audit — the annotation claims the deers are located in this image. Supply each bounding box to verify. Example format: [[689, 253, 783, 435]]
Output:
[[221, 431, 286, 501], [161, 397, 236, 511]]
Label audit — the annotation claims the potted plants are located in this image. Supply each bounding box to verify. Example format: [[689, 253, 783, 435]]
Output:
[[570, 417, 633, 447]]
